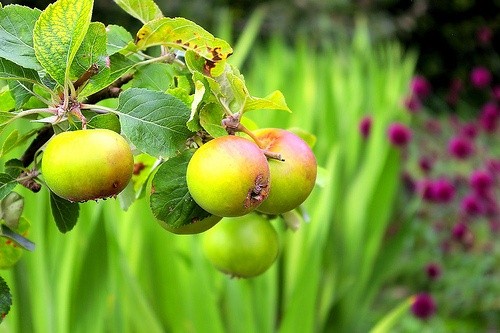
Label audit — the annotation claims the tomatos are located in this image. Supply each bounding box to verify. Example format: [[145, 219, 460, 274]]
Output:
[[151, 127, 317, 279], [42, 129, 135, 202]]
